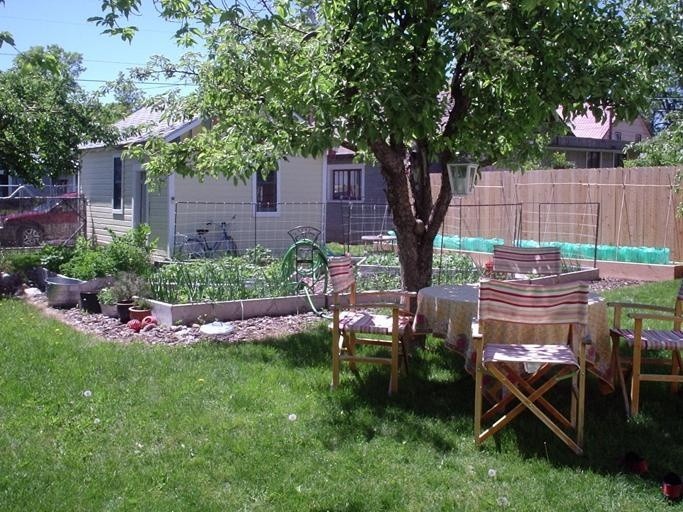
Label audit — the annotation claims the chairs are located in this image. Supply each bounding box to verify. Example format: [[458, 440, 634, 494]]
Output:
[[468, 267, 602, 452], [603, 263, 682, 420], [287, 224, 321, 293], [326, 250, 421, 397], [488, 246, 564, 282]]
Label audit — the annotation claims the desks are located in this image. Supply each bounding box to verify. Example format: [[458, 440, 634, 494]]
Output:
[[361, 234, 396, 253], [416, 285, 614, 417]]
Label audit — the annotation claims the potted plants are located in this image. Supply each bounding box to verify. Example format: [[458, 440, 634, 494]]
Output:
[[98, 277, 152, 326]]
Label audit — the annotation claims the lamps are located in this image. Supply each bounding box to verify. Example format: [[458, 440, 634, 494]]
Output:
[[448, 153, 479, 198]]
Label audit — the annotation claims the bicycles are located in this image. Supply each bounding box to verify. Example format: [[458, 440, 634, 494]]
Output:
[[179, 213, 240, 261]]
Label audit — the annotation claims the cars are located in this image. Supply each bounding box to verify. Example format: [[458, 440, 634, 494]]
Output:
[[0, 191, 77, 246]]
